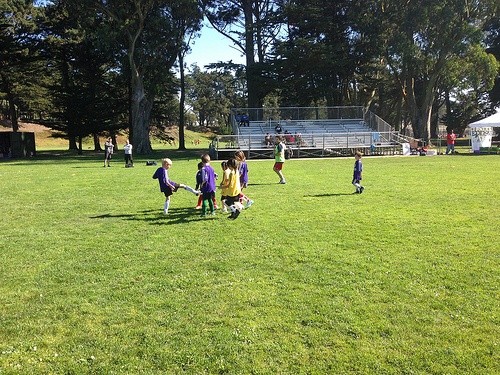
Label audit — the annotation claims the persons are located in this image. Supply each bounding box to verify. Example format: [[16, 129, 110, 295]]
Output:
[[446, 128, 456, 154], [265, 123, 302, 147], [152, 158, 201, 215], [235, 113, 242, 126], [352, 151, 364, 194], [468, 112, 500, 154], [123, 140, 133, 166], [195, 161, 220, 209], [243, 113, 249, 127], [273, 136, 286, 183], [216, 150, 254, 220], [104, 138, 114, 167], [200, 154, 216, 217]]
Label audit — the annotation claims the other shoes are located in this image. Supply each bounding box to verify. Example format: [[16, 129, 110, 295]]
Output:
[[279, 179, 285, 184], [214, 206, 217, 210], [196, 206, 201, 209], [195, 191, 201, 196], [221, 208, 226, 212], [360, 186, 364, 193], [353, 190, 359, 193], [200, 215, 205, 217], [211, 212, 215, 215], [163, 213, 169, 216]]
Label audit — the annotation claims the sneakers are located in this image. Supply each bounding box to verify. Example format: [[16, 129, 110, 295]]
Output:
[[245, 200, 254, 209], [232, 208, 240, 220], [228, 212, 234, 218]]
